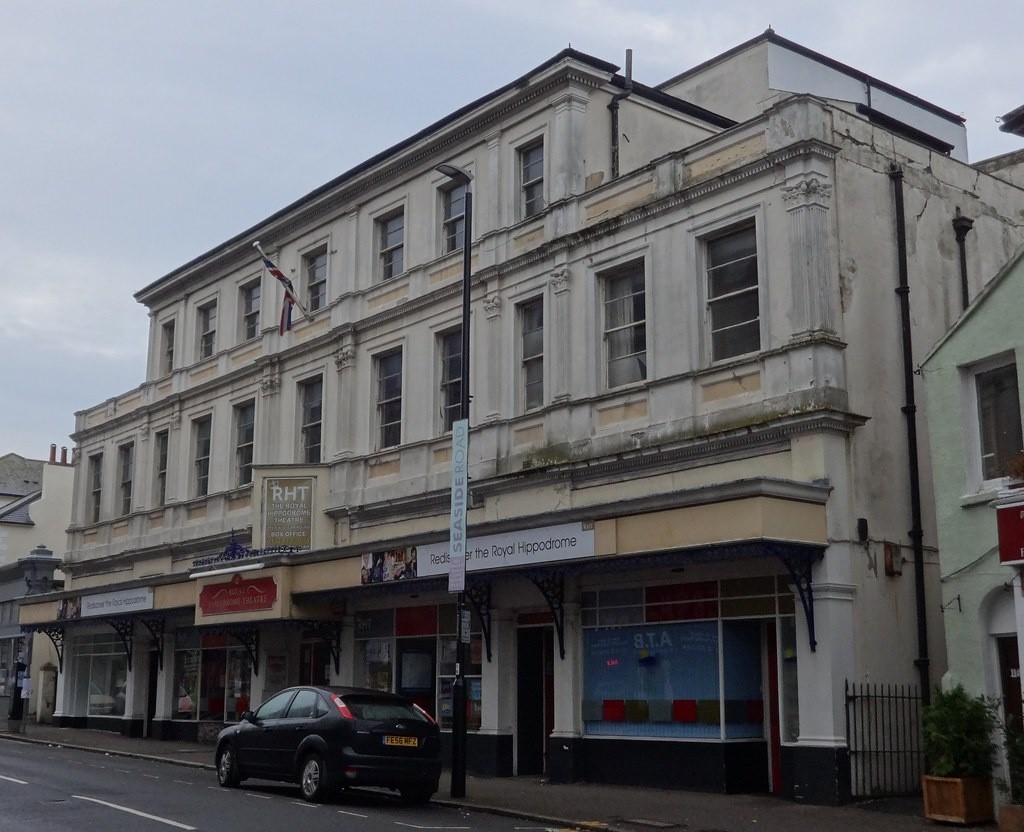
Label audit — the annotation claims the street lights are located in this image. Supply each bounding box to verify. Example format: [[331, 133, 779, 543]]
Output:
[[431, 164, 472, 803]]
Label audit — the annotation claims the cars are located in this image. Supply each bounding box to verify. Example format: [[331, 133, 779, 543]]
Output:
[[215, 687, 441, 800]]
